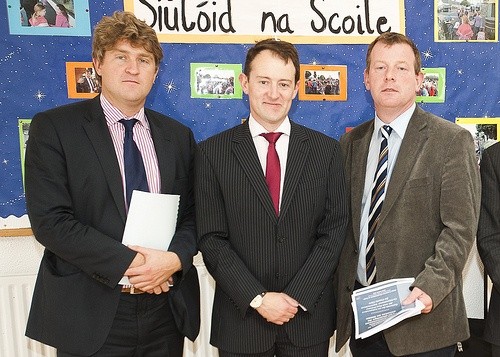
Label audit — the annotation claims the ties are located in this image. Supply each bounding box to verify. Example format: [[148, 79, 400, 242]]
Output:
[[118, 119, 150, 213], [366, 125, 393, 285], [258, 132, 285, 217]]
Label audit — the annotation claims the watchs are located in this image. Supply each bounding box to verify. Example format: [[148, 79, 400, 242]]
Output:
[[249, 292, 265, 311]]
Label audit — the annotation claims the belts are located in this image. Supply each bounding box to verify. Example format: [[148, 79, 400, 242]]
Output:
[[121, 285, 145, 294]]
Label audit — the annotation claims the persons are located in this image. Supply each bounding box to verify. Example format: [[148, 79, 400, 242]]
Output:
[[76, 68, 97, 94], [305, 76, 341, 95], [29, 0, 70, 28], [476, 142, 500, 357], [193, 39, 350, 357], [197, 75, 234, 95], [338, 34, 482, 357], [458, 4, 483, 42], [24, 9, 200, 357], [418, 78, 438, 96]]
